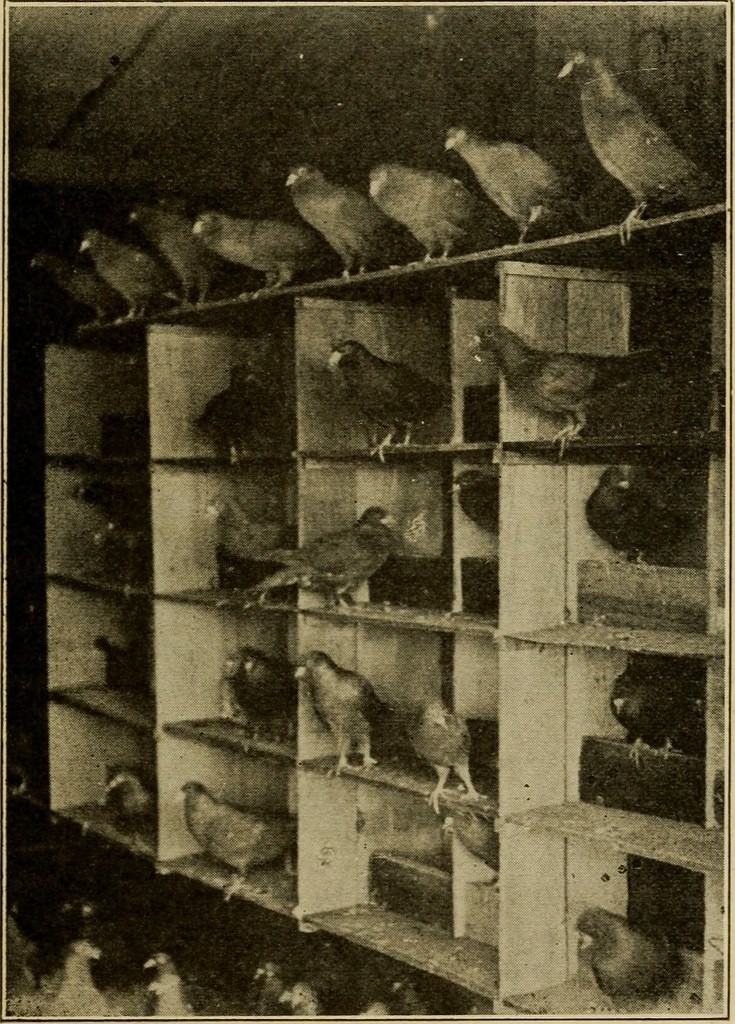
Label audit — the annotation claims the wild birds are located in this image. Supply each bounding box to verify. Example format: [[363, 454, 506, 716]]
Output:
[[332, 337, 445, 459], [583, 463, 689, 562], [125, 203, 221, 309], [612, 658, 710, 761], [553, 45, 723, 244], [189, 206, 317, 299], [574, 906, 693, 1017], [104, 762, 156, 838], [286, 162, 395, 271], [81, 232, 158, 329], [212, 641, 500, 804], [441, 126, 578, 253], [6, 907, 491, 1019], [183, 779, 298, 905], [366, 167, 483, 263], [21, 249, 121, 333], [439, 808, 513, 877]]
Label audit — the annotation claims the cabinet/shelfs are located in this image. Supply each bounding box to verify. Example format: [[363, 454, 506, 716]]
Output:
[[43, 206, 725, 1014]]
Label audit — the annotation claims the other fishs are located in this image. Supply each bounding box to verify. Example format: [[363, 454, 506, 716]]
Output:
[[228, 503, 398, 611], [469, 324, 668, 450]]
